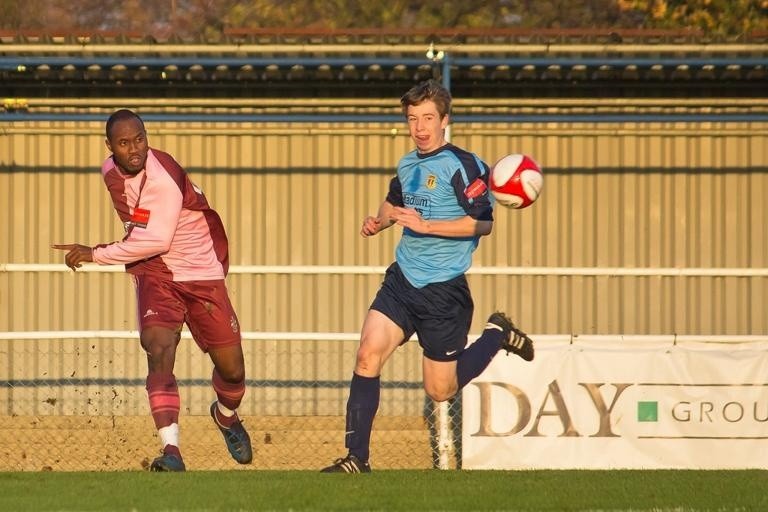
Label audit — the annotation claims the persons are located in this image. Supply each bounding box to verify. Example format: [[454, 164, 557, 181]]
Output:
[[317, 78, 535, 474], [50, 106, 254, 472]]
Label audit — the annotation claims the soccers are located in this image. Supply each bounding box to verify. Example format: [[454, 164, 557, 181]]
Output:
[[488, 153, 544, 209]]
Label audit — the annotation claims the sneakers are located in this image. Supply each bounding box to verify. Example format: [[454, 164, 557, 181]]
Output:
[[319, 455, 372, 474], [484, 312, 535, 362], [150, 454, 186, 473], [210, 401, 253, 465]]
